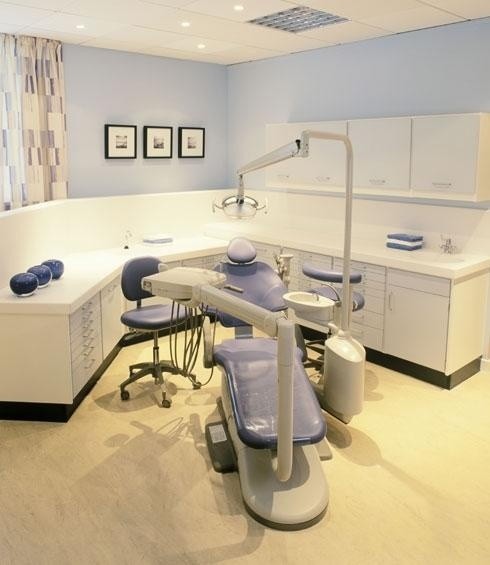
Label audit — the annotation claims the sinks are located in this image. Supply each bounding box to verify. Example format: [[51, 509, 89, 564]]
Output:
[[407, 251, 464, 264], [282, 289, 335, 315]]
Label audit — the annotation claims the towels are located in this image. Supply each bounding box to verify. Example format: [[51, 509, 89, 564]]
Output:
[[386, 234, 424, 251]]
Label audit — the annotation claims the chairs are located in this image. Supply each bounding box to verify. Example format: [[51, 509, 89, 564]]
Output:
[[199, 238, 328, 451]]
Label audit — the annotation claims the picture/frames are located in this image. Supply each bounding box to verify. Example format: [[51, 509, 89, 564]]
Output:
[[104, 124, 138, 158], [177, 127, 205, 157], [143, 125, 172, 159]]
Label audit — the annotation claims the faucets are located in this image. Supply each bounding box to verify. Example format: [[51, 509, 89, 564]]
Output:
[[438, 234, 455, 254], [319, 282, 339, 303], [123, 229, 133, 247]]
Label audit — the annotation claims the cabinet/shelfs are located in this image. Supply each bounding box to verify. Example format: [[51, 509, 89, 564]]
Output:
[[330, 256, 489, 394], [346, 116, 411, 201], [303, 120, 347, 198], [412, 113, 490, 204], [128, 240, 239, 338], [1, 240, 128, 421], [252, 240, 332, 349], [264, 123, 302, 193]]
